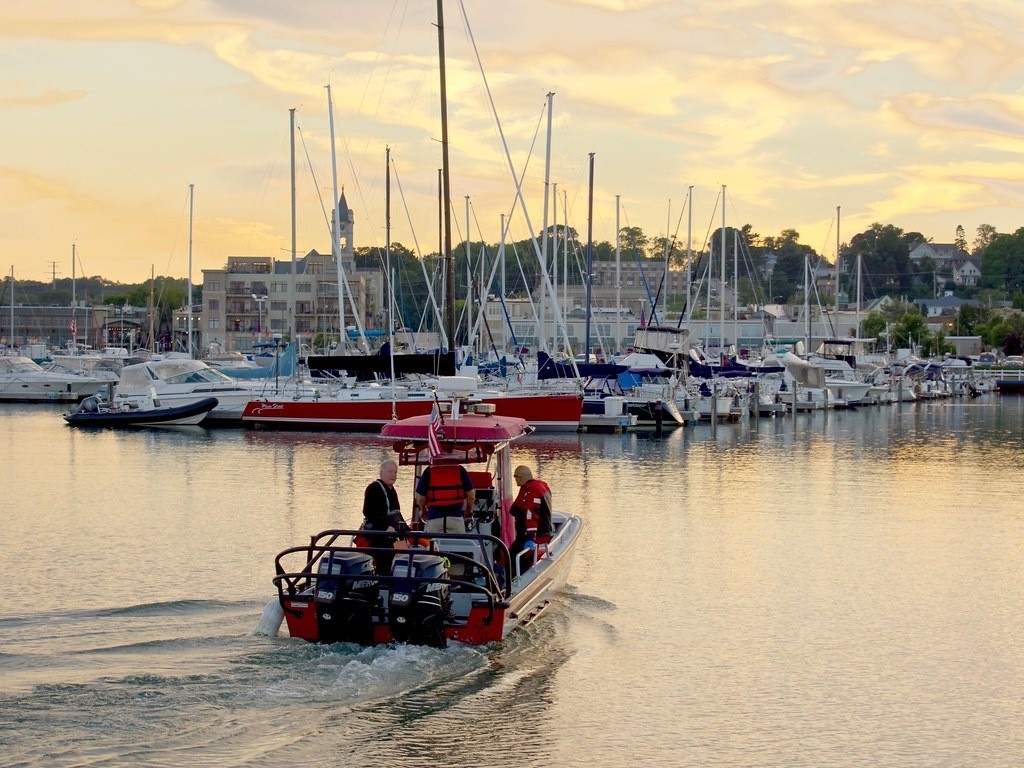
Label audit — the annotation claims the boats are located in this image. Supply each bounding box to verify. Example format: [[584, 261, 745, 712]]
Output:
[[272, 232, 585, 651], [62, 380, 221, 426]]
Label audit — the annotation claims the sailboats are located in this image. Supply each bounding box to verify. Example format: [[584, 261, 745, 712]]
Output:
[[0, 0, 1024, 440]]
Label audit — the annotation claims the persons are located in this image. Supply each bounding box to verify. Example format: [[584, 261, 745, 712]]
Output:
[[416, 464, 475, 533], [509, 465, 553, 577], [363, 460, 411, 577]]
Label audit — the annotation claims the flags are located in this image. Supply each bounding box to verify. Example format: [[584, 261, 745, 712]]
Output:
[[162, 337, 165, 348], [641, 310, 646, 327], [258, 318, 261, 333], [429, 397, 443, 464], [71, 319, 75, 335], [266, 326, 269, 336]]
